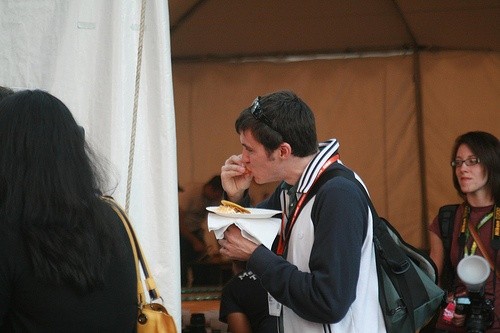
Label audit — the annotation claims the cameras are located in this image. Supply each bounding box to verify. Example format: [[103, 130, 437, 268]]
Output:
[[454, 255, 495, 333]]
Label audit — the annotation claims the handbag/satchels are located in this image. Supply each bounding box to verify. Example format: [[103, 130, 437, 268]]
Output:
[[317, 174, 447, 333], [136, 301, 179, 333]]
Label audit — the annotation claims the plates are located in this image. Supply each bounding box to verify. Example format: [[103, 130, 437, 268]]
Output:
[[205, 206, 283, 219]]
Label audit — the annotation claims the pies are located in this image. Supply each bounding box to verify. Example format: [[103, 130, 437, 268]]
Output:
[[221, 200, 251, 214]]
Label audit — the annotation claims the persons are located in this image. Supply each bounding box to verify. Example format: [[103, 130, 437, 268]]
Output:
[[219, 258, 279, 333], [428, 131, 500, 333], [178, 182, 194, 288], [217, 91, 388, 333], [188, 174, 238, 290], [0, 86, 141, 333]]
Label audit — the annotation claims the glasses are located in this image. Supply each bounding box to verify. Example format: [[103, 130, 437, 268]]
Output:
[[450, 157, 486, 167], [249, 95, 283, 136]]
